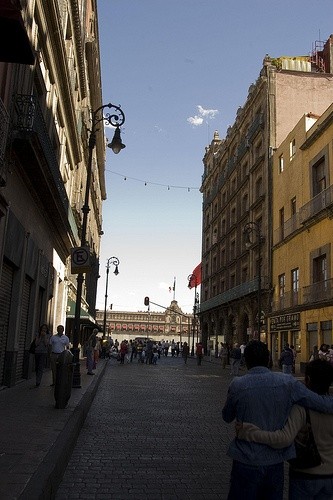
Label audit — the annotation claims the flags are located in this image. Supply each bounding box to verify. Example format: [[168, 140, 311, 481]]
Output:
[[187, 262, 202, 289]]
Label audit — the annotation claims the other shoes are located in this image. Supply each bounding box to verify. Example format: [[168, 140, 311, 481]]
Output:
[[50, 383, 55, 387], [36, 383, 40, 387]]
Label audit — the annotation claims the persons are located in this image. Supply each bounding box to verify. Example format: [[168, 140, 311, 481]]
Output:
[[229, 343, 242, 377], [48, 325, 71, 387], [28, 323, 51, 387], [308, 343, 333, 388], [279, 343, 294, 377], [93, 336, 102, 369], [86, 329, 98, 376], [233, 357, 333, 500], [288, 344, 297, 376], [221, 339, 333, 500], [98, 337, 247, 370]]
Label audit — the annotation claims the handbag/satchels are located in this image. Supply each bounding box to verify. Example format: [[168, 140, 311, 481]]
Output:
[[286, 403, 322, 470]]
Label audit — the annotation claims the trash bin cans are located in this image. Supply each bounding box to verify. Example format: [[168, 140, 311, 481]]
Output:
[[54, 349, 75, 410]]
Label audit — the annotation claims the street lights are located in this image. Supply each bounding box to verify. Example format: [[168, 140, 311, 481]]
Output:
[[242, 222, 264, 342], [144, 297, 149, 306], [67, 102, 127, 390], [100, 256, 120, 359], [186, 274, 200, 356]]
